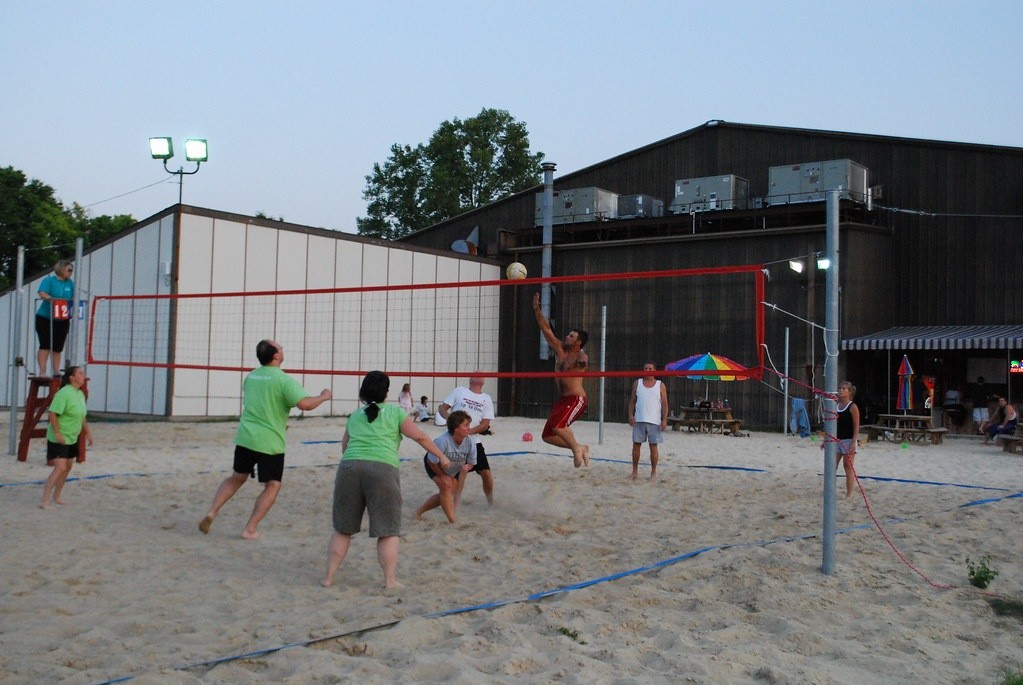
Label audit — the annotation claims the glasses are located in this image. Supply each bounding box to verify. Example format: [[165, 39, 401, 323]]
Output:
[[64, 268, 74, 272]]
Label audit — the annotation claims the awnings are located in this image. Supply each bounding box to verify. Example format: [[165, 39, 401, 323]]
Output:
[[841, 325, 1023, 351]]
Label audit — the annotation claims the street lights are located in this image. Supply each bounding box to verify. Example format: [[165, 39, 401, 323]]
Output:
[[149, 135, 208, 205]]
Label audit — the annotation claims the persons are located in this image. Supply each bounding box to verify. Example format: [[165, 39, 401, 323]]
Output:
[[320, 371, 451, 589], [440, 377, 494, 508], [944, 383, 968, 427], [821, 381, 860, 501], [532, 292, 589, 469], [199, 340, 333, 540], [986, 397, 1017, 442], [40, 367, 93, 509], [435, 412, 446, 426], [36, 260, 75, 378], [416, 411, 477, 525], [972, 376, 1000, 435], [629, 361, 669, 482], [398, 383, 414, 415], [414, 396, 429, 422]]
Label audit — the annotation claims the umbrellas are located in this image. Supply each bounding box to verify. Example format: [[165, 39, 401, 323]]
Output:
[[665, 352, 749, 401], [896, 354, 915, 428]]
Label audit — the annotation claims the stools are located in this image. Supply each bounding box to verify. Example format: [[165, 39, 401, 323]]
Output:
[[16, 377, 89, 463]]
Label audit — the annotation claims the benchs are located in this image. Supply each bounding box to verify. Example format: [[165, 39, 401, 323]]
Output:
[[997, 433, 1023, 442], [667, 416, 745, 425], [861, 425, 950, 435]]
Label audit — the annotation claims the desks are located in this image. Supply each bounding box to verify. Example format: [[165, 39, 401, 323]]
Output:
[[673, 406, 739, 433], [1010, 423, 1023, 453], [873, 414, 938, 445]]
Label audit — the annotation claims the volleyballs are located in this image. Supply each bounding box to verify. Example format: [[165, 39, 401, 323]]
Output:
[[506, 262, 527, 279], [522, 433, 533, 441]]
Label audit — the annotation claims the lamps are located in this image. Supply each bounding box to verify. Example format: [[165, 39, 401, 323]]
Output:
[[182, 138, 208, 175], [149, 136, 180, 175]]
